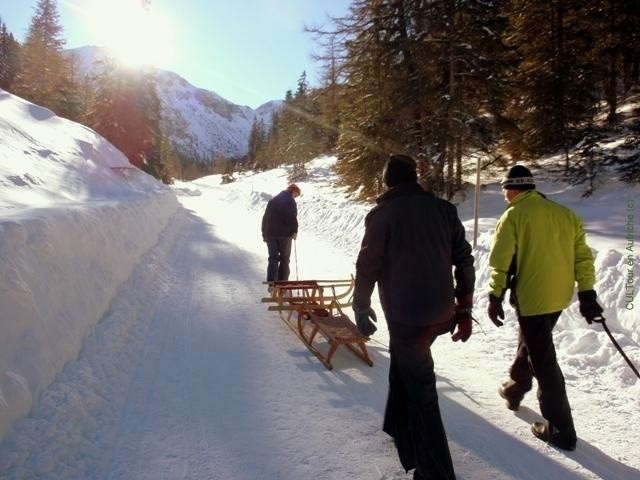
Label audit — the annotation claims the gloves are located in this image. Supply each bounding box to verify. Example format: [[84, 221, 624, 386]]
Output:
[[578, 290, 603, 324], [354, 303, 376, 336], [487, 294, 505, 327], [451, 312, 472, 342]]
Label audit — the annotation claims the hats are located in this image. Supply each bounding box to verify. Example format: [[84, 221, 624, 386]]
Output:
[[382, 154, 418, 184], [501, 165, 535, 191], [289, 184, 301, 195]]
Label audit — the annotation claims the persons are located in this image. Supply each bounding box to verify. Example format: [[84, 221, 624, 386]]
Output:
[[260, 182, 302, 293], [484, 164, 604, 453], [349, 152, 477, 480]]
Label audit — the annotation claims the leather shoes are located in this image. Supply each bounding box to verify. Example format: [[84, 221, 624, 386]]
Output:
[[498, 383, 520, 410], [530, 421, 576, 451]]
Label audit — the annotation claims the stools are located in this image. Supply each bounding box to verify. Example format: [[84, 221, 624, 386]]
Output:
[[297, 310, 372, 372]]
